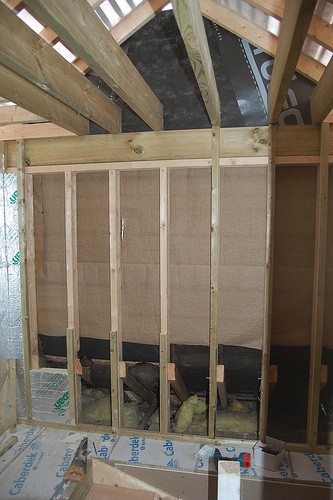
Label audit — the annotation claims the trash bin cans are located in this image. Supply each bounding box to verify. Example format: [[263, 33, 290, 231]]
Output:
[[256, 344, 331, 452]]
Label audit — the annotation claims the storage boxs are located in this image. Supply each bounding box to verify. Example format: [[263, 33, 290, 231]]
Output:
[[252, 435, 288, 471]]
